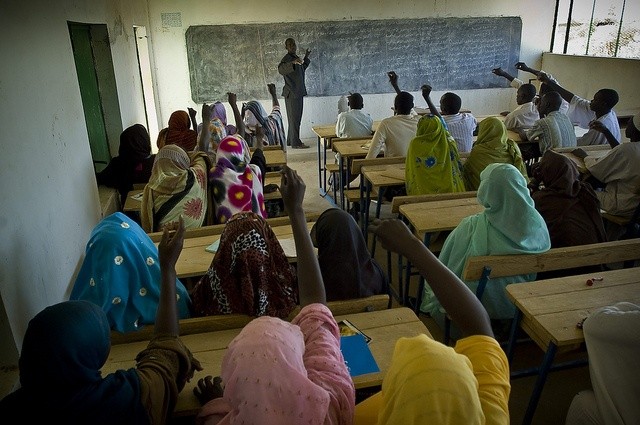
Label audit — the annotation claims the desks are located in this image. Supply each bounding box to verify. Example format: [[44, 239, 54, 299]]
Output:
[[360, 157, 469, 258], [99, 306, 437, 424], [557, 149, 613, 174], [332, 139, 384, 213], [507, 267, 639, 425], [475, 115, 506, 127], [250, 150, 287, 172], [153, 221, 321, 279], [311, 120, 381, 192], [507, 126, 589, 144], [123, 176, 283, 228], [398, 197, 487, 318]]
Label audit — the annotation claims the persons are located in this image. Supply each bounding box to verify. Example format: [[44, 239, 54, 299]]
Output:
[[440, 92, 478, 152], [511, 90, 577, 181], [404, 84, 474, 196], [99, 122, 156, 203], [492, 67, 541, 134], [197, 92, 245, 139], [420, 162, 552, 321], [310, 207, 394, 308], [67, 212, 192, 333], [209, 133, 267, 224], [239, 83, 281, 147], [570, 113, 640, 219], [351, 216, 512, 425], [564, 302, 638, 425], [156, 107, 198, 152], [1, 213, 193, 424], [535, 70, 621, 145], [278, 37, 312, 150], [514, 62, 569, 114], [188, 210, 296, 317], [527, 149, 608, 249], [464, 116, 533, 191], [365, 71, 422, 157], [193, 160, 354, 423], [141, 143, 209, 229], [328, 93, 373, 192]]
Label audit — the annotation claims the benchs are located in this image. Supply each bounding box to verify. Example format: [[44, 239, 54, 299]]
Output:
[[343, 152, 472, 236], [324, 135, 373, 204], [386, 191, 480, 307], [600, 205, 640, 268], [444, 238, 639, 347]]
